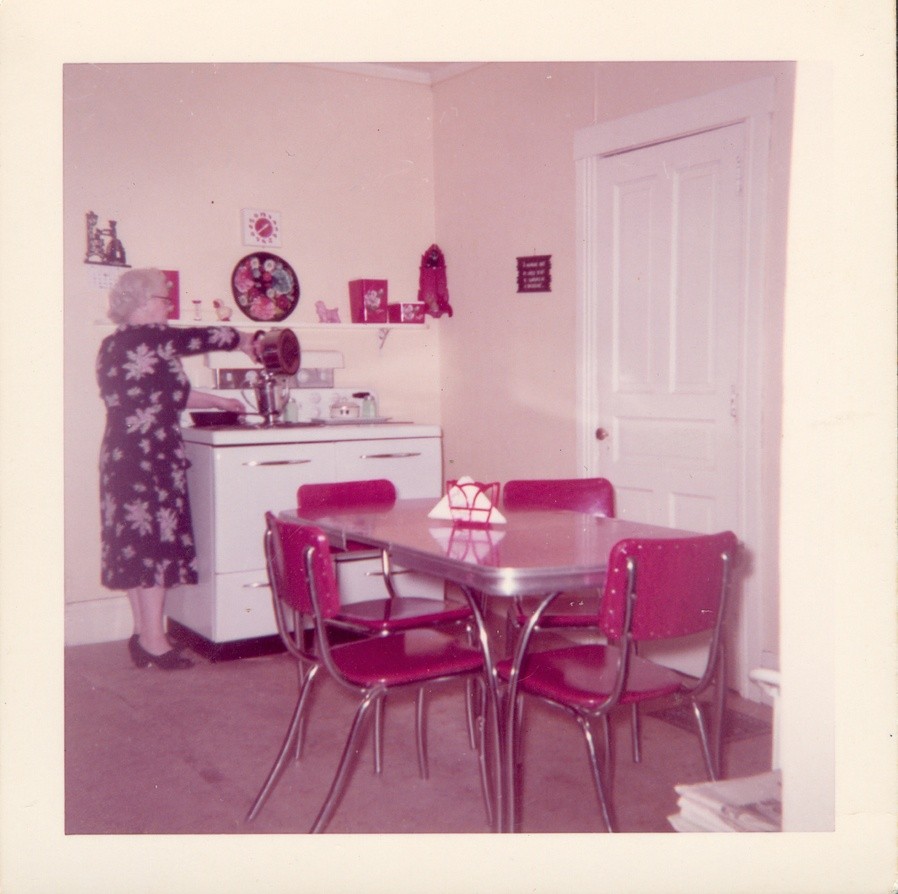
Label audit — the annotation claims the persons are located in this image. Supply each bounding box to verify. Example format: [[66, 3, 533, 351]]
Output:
[[94, 267, 262, 672]]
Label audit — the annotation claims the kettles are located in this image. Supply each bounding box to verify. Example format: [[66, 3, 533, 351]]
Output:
[[251, 328, 300, 379]]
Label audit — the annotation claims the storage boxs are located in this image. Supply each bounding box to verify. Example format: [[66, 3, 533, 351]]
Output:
[[349, 278, 387, 323], [388, 301, 426, 324]]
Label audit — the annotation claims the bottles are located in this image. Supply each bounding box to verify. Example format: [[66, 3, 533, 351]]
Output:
[[284, 399, 298, 424]]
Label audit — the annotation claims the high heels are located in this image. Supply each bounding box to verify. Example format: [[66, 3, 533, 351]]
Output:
[[135, 640, 192, 670], [128, 634, 188, 662]]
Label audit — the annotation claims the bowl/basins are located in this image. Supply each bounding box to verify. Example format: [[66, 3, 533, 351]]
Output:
[[331, 405, 360, 418], [190, 411, 247, 428]]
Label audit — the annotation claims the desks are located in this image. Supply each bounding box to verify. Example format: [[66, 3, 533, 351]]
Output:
[[278, 497, 743, 834]]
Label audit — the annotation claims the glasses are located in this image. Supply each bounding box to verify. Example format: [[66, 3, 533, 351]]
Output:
[[151, 296, 173, 304]]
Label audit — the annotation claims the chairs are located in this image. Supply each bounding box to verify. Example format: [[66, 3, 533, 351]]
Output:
[[244, 477, 738, 833]]
